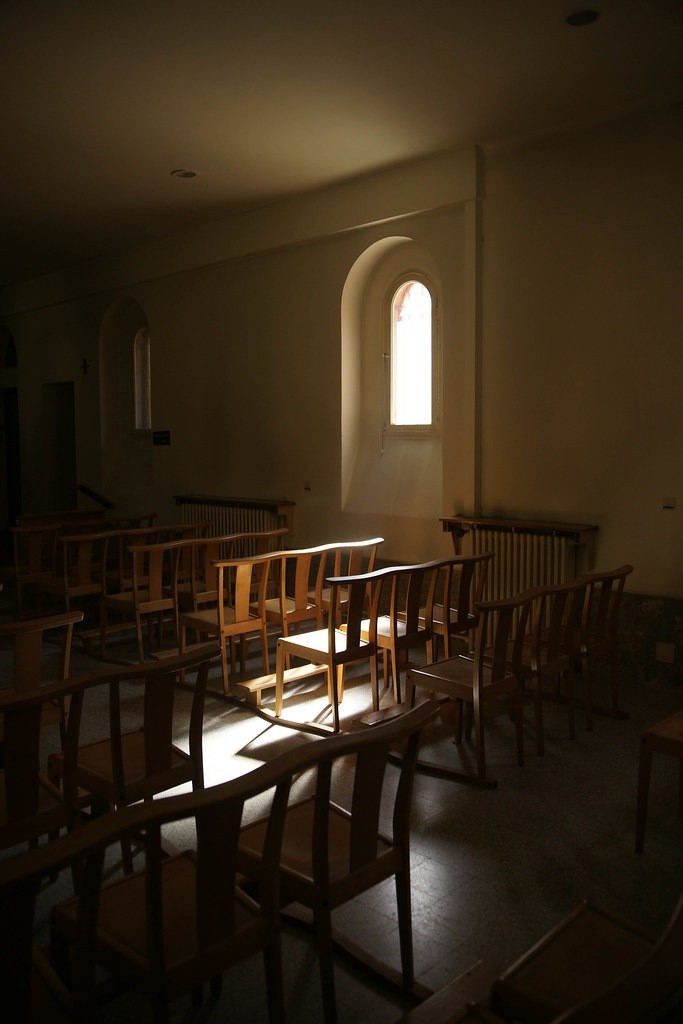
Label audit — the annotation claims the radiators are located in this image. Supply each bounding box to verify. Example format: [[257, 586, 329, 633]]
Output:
[[469, 529, 576, 639], [181, 502, 277, 587]]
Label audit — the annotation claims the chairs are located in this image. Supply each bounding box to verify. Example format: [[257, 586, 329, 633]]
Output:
[[0, 611, 87, 752], [0, 513, 158, 619], [634, 706, 683, 853], [393, 897, 683, 1024], [180, 537, 385, 694], [405, 564, 633, 789], [274, 552, 494, 733], [0, 698, 437, 1024], [0, 644, 222, 895], [99, 528, 292, 666], [35, 521, 201, 640]]
